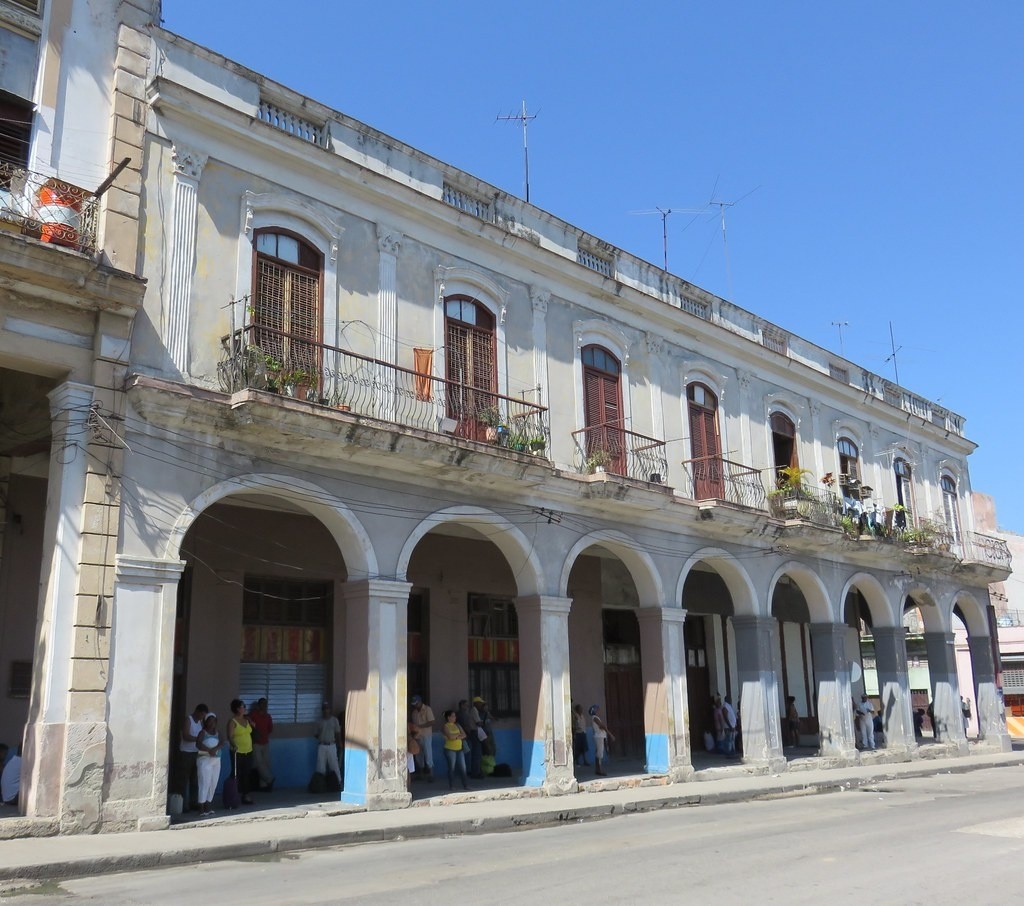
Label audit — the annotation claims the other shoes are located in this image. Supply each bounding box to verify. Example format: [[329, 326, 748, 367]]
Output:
[[208, 810, 215, 814], [200, 811, 208, 817]]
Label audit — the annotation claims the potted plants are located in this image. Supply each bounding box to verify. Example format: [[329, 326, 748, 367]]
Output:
[[475, 403, 546, 454], [900, 508, 952, 552], [584, 449, 612, 475], [244, 346, 352, 410], [767, 467, 817, 520], [0, 207, 41, 238]]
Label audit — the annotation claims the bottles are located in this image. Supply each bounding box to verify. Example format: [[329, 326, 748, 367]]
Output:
[[170, 794, 183, 814]]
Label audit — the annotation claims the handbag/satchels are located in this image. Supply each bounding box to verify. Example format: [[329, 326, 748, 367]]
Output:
[[407, 722, 420, 755], [476, 724, 487, 742]]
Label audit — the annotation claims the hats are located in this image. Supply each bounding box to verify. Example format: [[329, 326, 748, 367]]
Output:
[[410, 695, 422, 705], [589, 704, 599, 715], [861, 693, 869, 697], [473, 697, 487, 703]]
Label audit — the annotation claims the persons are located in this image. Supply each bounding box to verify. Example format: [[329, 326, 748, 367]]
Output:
[[0, 743, 24, 807], [852, 695, 884, 751], [960, 696, 971, 738], [571, 698, 616, 776], [913, 709, 925, 737], [407, 695, 497, 792], [313, 700, 343, 789], [927, 701, 936, 737], [786, 695, 800, 749], [711, 695, 741, 755], [184, 697, 274, 817]]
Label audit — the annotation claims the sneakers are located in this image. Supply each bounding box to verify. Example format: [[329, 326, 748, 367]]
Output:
[[241, 796, 252, 804]]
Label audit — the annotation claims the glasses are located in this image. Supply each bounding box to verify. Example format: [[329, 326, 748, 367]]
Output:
[[239, 705, 246, 708]]
